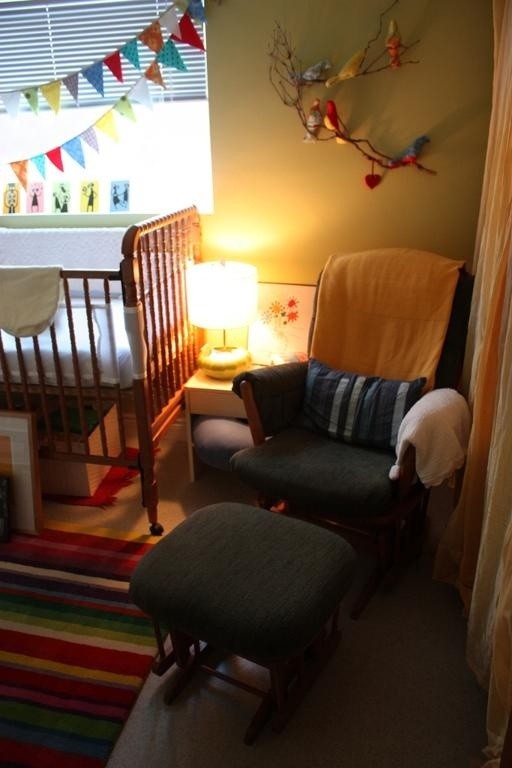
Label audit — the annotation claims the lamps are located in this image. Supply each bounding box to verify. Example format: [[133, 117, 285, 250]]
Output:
[[187, 260, 258, 380]]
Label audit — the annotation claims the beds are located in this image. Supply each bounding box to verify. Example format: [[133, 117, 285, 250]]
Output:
[[0, 205, 205, 537]]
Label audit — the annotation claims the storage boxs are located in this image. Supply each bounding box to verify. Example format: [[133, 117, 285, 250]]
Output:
[[15, 400, 125, 498]]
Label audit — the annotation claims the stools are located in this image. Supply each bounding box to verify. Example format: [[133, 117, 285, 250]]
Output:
[[130, 502, 364, 745]]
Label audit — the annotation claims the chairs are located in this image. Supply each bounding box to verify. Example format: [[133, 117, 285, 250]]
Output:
[[228, 248, 475, 621]]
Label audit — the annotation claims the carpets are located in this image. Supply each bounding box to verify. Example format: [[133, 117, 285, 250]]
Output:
[[0, 521, 170, 768], [41, 445, 161, 511]]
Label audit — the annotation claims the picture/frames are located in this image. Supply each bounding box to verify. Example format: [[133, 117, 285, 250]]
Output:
[[248, 281, 318, 366], [0, 411, 43, 535]]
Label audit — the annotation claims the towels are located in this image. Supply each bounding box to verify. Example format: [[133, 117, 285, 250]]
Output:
[[390, 388, 473, 490]]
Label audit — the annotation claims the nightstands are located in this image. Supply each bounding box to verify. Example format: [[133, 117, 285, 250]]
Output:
[[182, 364, 262, 485]]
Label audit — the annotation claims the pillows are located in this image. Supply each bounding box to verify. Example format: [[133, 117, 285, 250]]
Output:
[[301, 359, 427, 452], [193, 416, 250, 469]]
